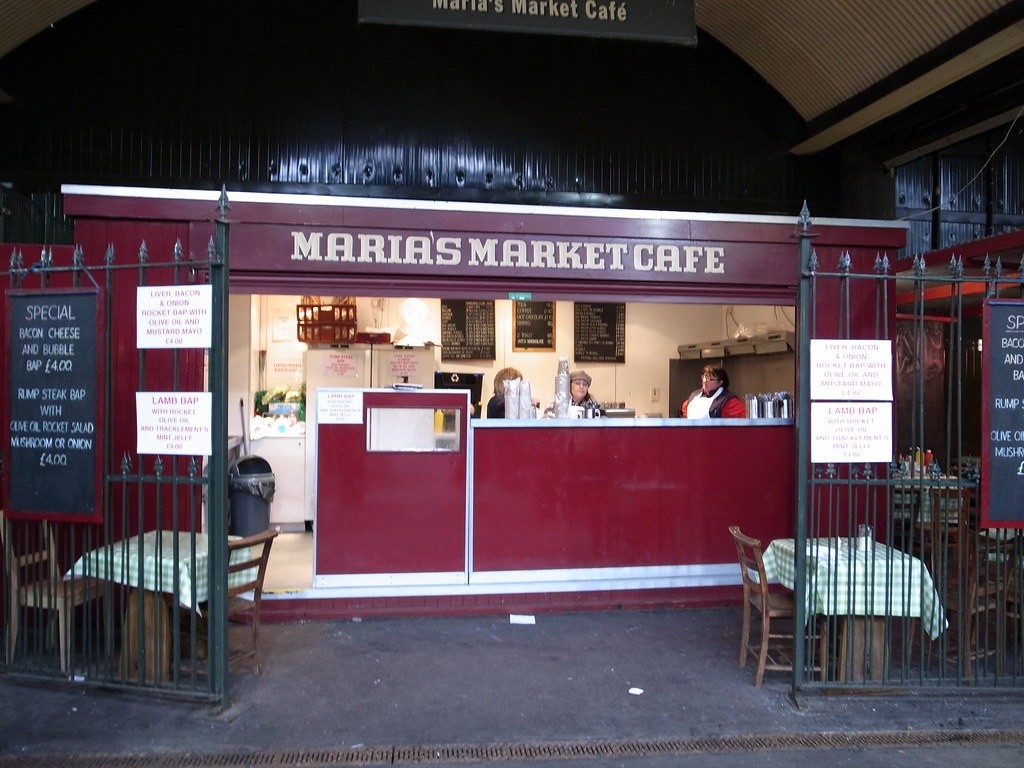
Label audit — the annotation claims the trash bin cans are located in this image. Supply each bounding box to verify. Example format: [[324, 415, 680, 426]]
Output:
[[227, 455, 276, 538]]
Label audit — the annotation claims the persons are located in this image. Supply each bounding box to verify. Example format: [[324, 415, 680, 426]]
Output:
[[678, 363, 745, 418], [487, 367, 539, 419], [570, 370, 607, 417]]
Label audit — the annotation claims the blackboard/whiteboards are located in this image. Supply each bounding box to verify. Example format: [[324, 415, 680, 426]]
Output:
[[3, 288, 106, 524], [982, 297, 1023, 528]]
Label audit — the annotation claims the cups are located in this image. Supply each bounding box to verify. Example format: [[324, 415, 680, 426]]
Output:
[[504, 379, 531, 419], [554, 357, 570, 418]]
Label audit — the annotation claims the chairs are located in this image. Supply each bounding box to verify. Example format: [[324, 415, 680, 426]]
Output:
[[1, 511, 112, 675], [728, 525, 830, 687], [167, 531, 277, 675], [895, 487, 1024, 680]]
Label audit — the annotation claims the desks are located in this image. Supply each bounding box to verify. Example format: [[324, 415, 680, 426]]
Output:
[[61, 529, 251, 682], [755, 537, 948, 681]]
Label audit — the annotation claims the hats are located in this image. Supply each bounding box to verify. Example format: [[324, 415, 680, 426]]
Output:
[[569, 371, 592, 386]]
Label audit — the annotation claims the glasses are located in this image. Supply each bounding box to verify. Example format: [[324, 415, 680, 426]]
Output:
[[701, 375, 719, 382]]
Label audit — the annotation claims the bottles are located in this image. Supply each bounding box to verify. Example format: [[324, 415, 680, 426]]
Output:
[[859, 523, 873, 552], [897, 446, 934, 475]]
